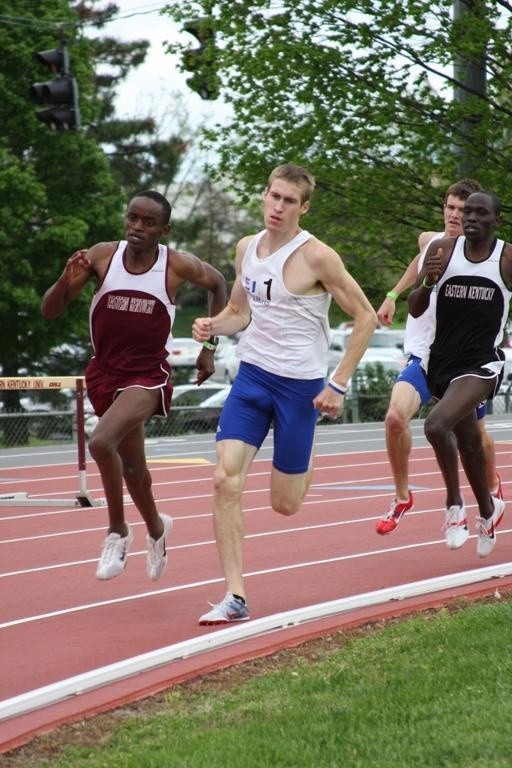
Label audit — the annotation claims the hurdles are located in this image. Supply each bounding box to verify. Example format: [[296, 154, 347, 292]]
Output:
[[0, 376, 154, 508]]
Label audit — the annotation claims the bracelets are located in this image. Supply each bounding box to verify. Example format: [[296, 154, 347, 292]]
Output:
[[327, 380, 349, 395], [422, 275, 438, 290], [203, 341, 218, 351], [207, 334, 219, 345], [385, 291, 403, 302]]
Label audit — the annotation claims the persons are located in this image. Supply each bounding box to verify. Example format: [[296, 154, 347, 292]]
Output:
[[40, 188, 228, 585], [375, 176, 505, 536], [406, 190, 512, 560], [189, 162, 378, 626]]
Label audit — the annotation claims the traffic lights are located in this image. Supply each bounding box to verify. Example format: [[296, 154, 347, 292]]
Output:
[[27, 49, 75, 131], [183, 15, 220, 102]]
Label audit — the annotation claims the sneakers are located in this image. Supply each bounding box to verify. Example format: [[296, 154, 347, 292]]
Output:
[[473, 494, 506, 560], [145, 511, 174, 584], [442, 492, 466, 552], [374, 490, 415, 537], [197, 592, 251, 627], [491, 470, 505, 532], [95, 521, 135, 582]]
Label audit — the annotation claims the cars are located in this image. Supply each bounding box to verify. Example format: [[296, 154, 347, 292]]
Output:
[[18, 327, 411, 437]]
